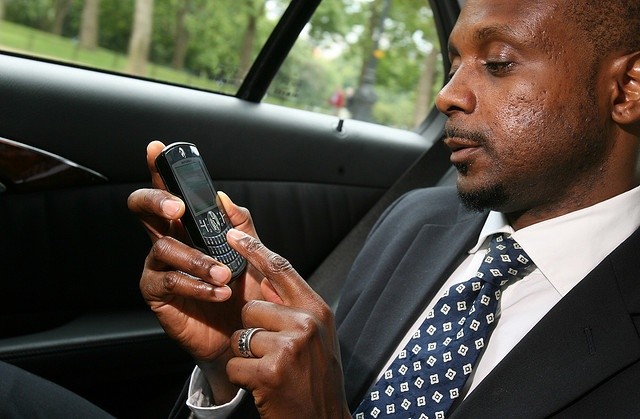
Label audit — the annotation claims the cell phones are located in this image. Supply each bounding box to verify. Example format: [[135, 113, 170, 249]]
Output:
[[154, 139, 248, 284]]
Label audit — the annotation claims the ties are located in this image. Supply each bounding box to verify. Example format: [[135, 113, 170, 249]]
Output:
[[351, 232, 535, 419]]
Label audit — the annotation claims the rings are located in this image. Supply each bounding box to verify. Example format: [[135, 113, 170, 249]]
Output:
[[237, 327, 264, 359]]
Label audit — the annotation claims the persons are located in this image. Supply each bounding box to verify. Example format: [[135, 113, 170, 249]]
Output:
[[128, 2, 638, 418]]
